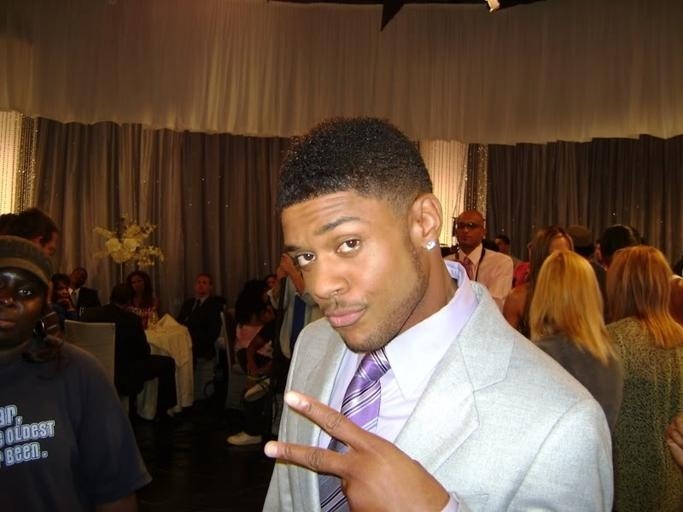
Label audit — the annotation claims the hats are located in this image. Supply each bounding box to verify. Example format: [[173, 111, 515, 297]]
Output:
[[2, 234, 53, 286]]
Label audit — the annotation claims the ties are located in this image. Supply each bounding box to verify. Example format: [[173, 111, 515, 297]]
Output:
[[463, 256, 472, 279], [290, 296, 306, 356], [319, 347, 390, 511]]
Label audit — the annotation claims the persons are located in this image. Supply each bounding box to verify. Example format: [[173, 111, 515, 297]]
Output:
[[263, 116, 614, 512], [440, 210, 682, 511], [2, 208, 330, 512]]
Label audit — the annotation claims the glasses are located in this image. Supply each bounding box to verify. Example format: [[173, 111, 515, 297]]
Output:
[[457, 222, 477, 230]]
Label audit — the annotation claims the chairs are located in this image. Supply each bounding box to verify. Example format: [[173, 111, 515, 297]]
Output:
[[192, 304, 227, 401], [221, 308, 273, 409], [64, 320, 117, 397]]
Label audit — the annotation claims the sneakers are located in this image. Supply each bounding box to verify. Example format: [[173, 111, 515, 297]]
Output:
[[245, 379, 271, 401], [227, 431, 262, 446]]
